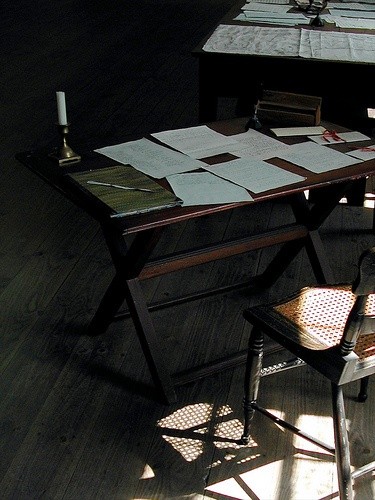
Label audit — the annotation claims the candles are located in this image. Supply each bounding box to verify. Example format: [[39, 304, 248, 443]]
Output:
[[55, 90, 67, 125]]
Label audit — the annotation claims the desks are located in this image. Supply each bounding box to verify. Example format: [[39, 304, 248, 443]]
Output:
[[13, 114, 375, 405], [192, 0, 375, 208]]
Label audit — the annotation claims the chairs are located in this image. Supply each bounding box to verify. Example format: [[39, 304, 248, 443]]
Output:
[[240, 244, 375, 500], [251, 88, 323, 128]]
